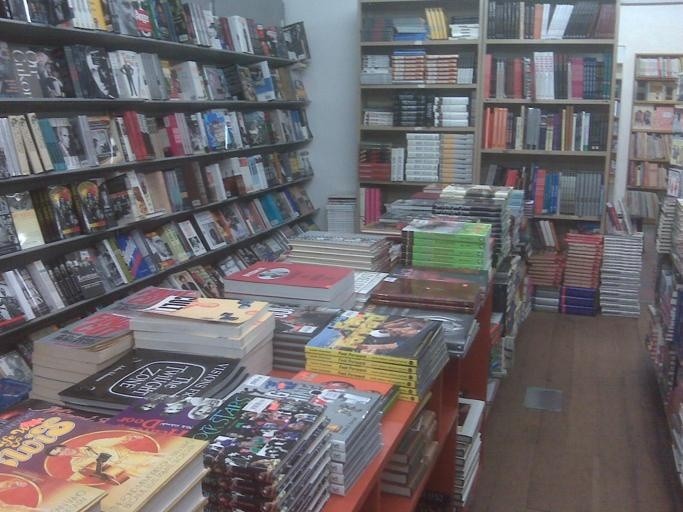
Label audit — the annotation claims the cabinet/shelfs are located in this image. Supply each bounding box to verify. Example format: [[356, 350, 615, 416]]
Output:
[[357, 3, 483, 232], [0, 16, 321, 349], [622, 52, 678, 240], [478, 2, 619, 252], [321, 264, 501, 511], [633, 221, 682, 489]]
